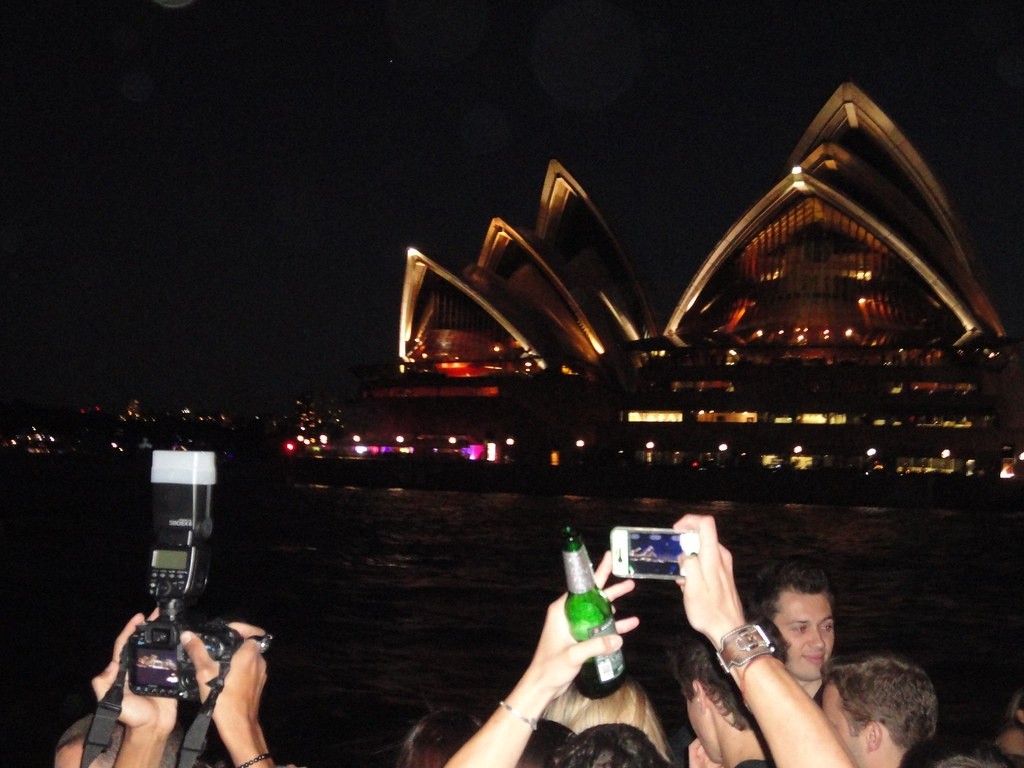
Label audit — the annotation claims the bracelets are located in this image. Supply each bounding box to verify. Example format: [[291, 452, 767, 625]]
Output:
[[498, 699, 537, 733], [237, 751, 271, 768], [715, 622, 786, 679]]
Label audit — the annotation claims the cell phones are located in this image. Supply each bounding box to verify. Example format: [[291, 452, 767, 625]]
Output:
[[610, 527, 701, 580]]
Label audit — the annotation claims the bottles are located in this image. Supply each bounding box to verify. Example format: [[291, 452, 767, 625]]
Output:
[[559, 526, 627, 699]]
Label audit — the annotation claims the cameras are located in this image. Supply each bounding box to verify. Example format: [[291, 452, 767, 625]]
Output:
[[127, 450, 244, 699]]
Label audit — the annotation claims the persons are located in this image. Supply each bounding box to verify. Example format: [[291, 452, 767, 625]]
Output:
[[659, 624, 775, 768], [439, 510, 860, 768], [744, 552, 835, 710], [392, 662, 677, 768], [51, 711, 187, 768], [819, 650, 1024, 768], [91, 605, 294, 768]]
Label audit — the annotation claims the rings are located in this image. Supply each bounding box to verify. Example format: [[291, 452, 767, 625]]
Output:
[[245, 634, 273, 653]]
